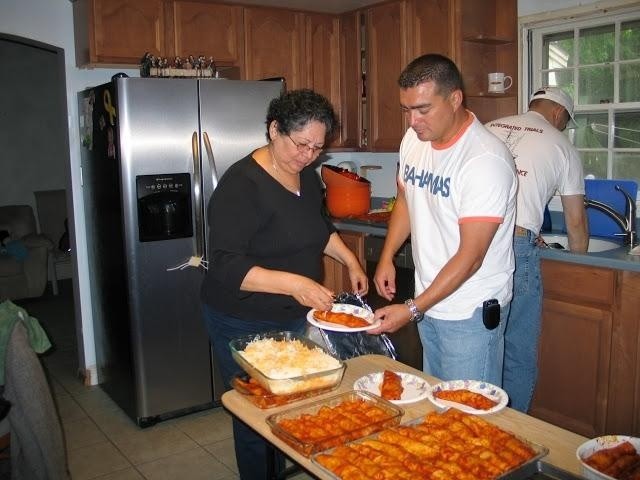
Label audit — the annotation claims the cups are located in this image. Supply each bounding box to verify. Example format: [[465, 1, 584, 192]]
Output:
[[486, 71, 514, 94]]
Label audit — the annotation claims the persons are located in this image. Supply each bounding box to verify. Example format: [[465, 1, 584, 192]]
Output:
[[196, 88, 372, 480], [139, 51, 218, 79], [363, 51, 520, 406], [482, 85, 591, 415]]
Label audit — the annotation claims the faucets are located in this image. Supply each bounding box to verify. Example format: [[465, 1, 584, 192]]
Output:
[[584, 184, 637, 245]]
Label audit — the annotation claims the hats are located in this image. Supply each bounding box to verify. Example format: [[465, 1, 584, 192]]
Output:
[[529, 87, 579, 129]]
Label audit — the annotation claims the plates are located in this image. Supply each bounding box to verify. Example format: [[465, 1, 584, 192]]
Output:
[[574, 434, 640, 479], [305, 303, 382, 335], [352, 370, 430, 407], [427, 379, 510, 416]]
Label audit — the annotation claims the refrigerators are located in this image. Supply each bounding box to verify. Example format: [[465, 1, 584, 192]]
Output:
[[75, 72, 286, 425]]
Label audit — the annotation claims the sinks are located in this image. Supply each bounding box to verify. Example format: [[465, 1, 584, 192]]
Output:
[[541, 235, 620, 253]]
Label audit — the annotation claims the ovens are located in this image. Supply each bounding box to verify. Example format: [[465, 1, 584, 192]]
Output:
[[366, 260, 422, 370]]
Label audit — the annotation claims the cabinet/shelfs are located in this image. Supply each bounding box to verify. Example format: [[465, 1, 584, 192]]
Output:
[[86, 0, 243, 68], [348, 0, 405, 153], [319, 230, 365, 298], [527, 257, 640, 440], [243, 4, 348, 149], [404, 0, 518, 140]]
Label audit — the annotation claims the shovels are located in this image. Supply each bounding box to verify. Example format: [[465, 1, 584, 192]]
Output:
[[537, 235, 565, 250]]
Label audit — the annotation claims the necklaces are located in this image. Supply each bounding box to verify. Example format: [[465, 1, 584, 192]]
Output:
[[266, 147, 303, 198]]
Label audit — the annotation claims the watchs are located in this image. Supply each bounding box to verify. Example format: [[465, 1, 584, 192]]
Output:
[[404, 297, 424, 323]]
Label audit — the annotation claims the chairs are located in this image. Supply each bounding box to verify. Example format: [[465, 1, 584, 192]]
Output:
[[34, 188, 74, 296]]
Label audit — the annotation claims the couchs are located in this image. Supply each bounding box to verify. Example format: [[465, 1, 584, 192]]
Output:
[[0, 204, 50, 301]]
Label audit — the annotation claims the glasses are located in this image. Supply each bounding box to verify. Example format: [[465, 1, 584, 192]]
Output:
[[288, 134, 323, 154]]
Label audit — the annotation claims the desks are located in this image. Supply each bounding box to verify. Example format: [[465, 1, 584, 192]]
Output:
[[221, 353, 640, 480]]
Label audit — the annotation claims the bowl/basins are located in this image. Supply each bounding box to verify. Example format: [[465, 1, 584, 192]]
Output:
[[322, 164, 371, 220]]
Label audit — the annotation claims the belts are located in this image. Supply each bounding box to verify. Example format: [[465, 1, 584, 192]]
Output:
[[513, 226, 527, 237]]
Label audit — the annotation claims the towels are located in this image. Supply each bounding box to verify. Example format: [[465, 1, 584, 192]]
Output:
[[1, 302, 51, 387]]
